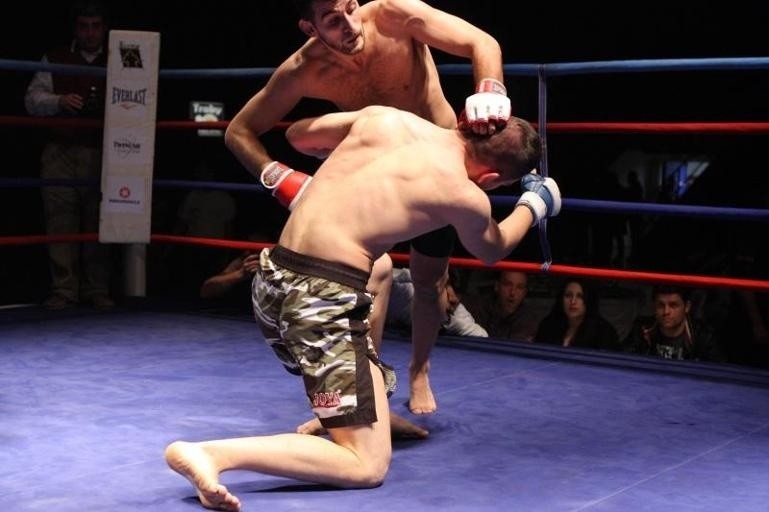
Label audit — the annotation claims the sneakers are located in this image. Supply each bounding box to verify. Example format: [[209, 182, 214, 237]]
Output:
[[40, 294, 75, 310], [87, 292, 115, 312]]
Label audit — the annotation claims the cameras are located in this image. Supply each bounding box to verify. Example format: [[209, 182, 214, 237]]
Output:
[[74, 86, 107, 119]]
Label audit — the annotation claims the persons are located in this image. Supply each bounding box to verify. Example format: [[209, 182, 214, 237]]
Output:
[[220, 0, 515, 435], [195, 234, 261, 310], [24, 1, 768, 369], [167, 104, 567, 511]]
